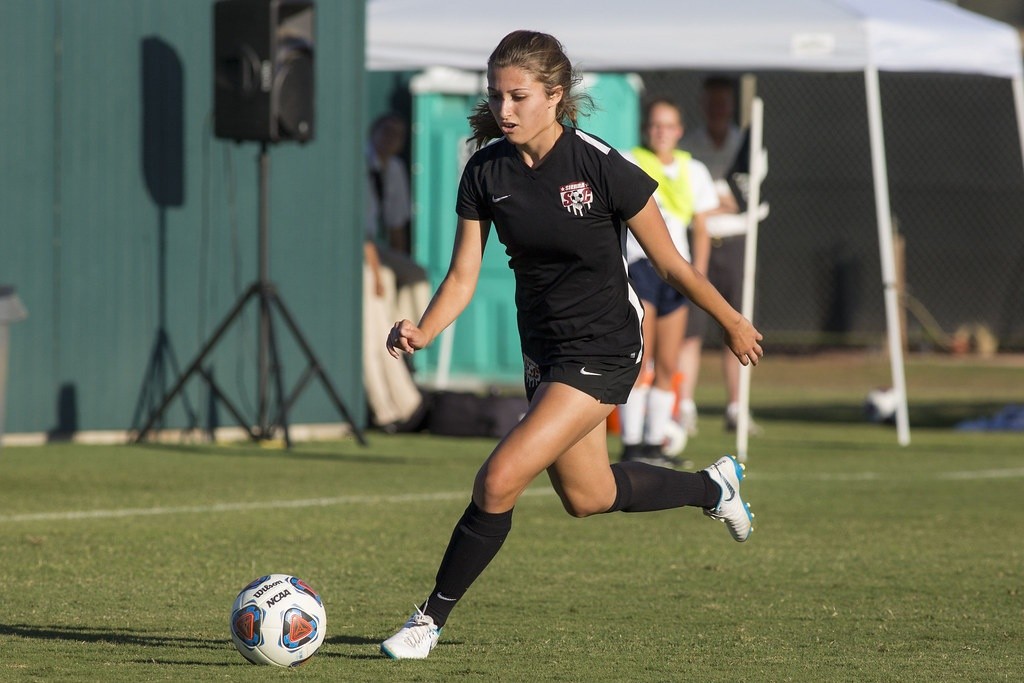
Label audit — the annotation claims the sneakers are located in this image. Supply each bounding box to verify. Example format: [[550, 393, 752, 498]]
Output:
[[701, 454, 755, 543], [380, 596, 446, 662]]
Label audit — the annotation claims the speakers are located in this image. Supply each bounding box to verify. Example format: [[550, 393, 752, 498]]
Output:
[[211, 0, 318, 145]]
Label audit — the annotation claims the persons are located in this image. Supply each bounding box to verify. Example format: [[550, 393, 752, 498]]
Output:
[[380, 30, 764, 660], [620, 95, 769, 462], [361, 113, 433, 432]]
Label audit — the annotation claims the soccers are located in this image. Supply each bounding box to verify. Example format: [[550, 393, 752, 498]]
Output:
[[228, 572, 329, 670]]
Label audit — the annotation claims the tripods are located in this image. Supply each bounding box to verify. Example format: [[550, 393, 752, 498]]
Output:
[[135, 143, 368, 449]]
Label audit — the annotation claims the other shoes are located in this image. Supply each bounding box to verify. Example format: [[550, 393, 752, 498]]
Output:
[[677, 411, 699, 437], [619, 444, 682, 465], [725, 403, 762, 433], [659, 430, 689, 458]]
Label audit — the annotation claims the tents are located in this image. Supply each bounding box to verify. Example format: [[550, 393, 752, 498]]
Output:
[[280, 0, 1024, 442]]
